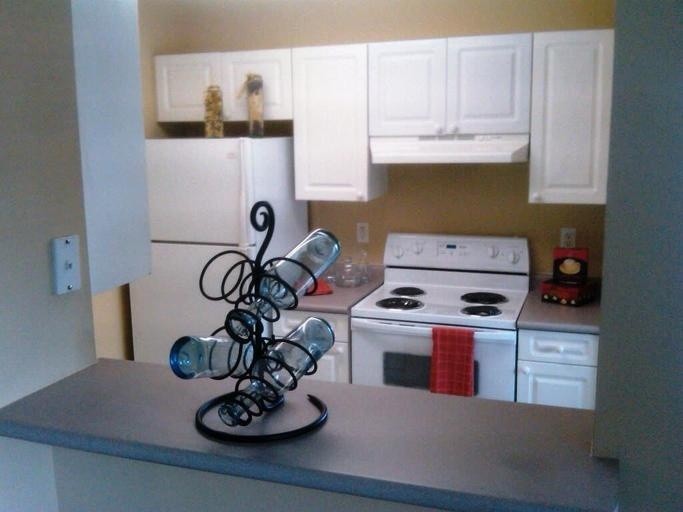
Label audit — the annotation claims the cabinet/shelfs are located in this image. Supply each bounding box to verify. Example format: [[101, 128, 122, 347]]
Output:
[[292, 43, 387, 203], [153, 48, 293, 122], [366, 32, 532, 135], [272, 307, 350, 384], [528, 28, 614, 206], [516, 328, 600, 411]]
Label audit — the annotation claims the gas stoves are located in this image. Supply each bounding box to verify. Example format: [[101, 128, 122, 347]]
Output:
[[351, 267, 529, 331]]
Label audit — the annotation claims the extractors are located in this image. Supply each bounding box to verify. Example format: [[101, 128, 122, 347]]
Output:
[[369, 136, 530, 167]]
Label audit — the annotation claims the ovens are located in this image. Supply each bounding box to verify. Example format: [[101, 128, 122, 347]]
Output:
[[351, 317, 515, 401]]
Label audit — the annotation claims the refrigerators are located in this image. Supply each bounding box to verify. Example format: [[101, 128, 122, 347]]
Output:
[[130, 136, 309, 364]]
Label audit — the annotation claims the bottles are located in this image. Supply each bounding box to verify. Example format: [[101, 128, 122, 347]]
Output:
[[203, 82, 222, 140], [247, 74, 264, 138], [326, 248, 376, 288], [216, 315, 335, 426], [233, 228, 341, 333], [169, 333, 269, 379]]
